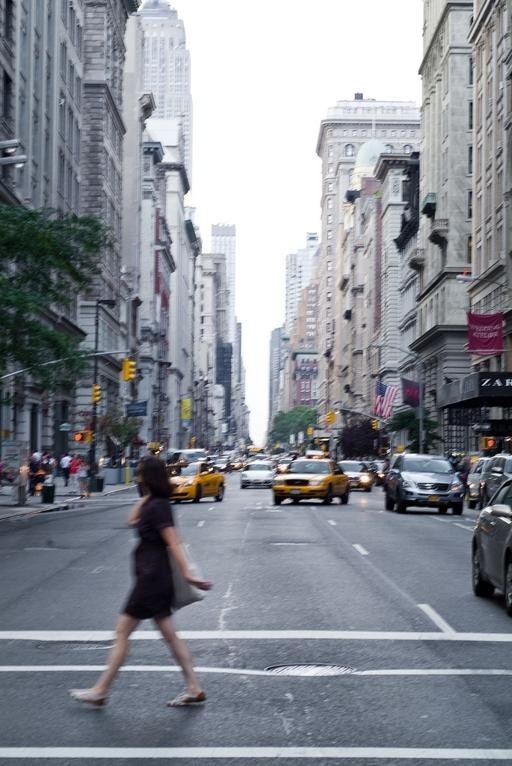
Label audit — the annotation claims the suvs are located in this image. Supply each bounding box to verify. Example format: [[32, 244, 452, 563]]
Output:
[[383, 451, 467, 515], [478, 450, 512, 509]]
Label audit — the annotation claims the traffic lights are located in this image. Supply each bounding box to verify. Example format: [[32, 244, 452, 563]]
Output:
[[479, 434, 501, 452], [90, 382, 102, 404], [122, 357, 138, 382], [73, 430, 87, 441], [326, 411, 332, 424], [371, 419, 376, 429]]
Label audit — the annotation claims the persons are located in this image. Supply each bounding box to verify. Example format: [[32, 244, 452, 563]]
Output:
[[225, 456, 232, 473], [25, 448, 117, 499], [66, 456, 213, 706], [382, 459, 391, 492]]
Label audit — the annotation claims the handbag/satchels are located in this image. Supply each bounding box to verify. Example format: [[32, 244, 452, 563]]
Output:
[[170, 555, 203, 609]]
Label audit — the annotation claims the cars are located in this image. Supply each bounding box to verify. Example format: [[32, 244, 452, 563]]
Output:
[[269, 448, 352, 505], [161, 458, 226, 504], [154, 442, 389, 493], [466, 455, 491, 508], [468, 476, 512, 616]]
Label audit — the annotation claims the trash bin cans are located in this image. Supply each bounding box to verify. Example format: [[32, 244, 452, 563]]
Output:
[[42, 484, 55, 503]]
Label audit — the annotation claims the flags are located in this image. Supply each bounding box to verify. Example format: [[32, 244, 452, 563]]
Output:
[[370, 381, 399, 420], [400, 378, 419, 409], [466, 311, 504, 356]]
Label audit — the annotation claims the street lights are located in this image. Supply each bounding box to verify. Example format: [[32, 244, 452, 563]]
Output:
[[81, 296, 116, 492], [371, 341, 426, 457], [0, 152, 30, 170], [455, 272, 512, 293], [0, 138, 20, 155]]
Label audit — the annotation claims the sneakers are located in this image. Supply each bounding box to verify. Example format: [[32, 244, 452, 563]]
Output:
[[169, 690, 207, 707], [69, 688, 109, 706]]
